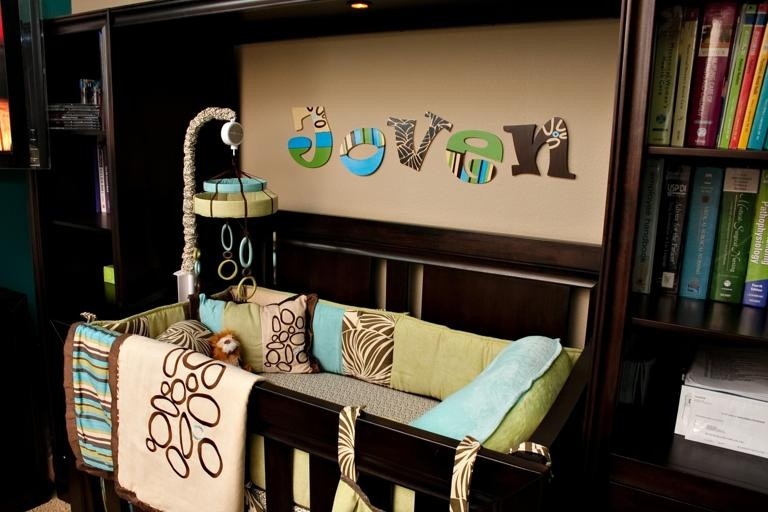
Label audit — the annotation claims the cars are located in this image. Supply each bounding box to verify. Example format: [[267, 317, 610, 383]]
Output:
[[78, 78, 102, 106]]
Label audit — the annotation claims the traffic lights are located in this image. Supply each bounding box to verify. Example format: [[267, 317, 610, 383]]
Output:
[[198, 290, 325, 375]]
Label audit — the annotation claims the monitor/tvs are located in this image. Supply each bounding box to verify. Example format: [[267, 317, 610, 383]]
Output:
[[92, 137, 112, 216]]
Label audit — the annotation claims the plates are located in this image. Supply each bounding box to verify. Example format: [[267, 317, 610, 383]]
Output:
[[568, 3, 768, 512], [16, 11, 232, 504]]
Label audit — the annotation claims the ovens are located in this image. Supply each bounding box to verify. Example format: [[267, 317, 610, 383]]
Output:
[[62, 284, 598, 511]]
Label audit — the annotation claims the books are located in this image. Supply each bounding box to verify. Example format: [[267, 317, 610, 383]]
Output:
[[633, 156, 664, 294], [679, 166, 723, 300], [709, 168, 761, 303], [656, 165, 691, 294], [92, 137, 112, 216], [646, 7, 765, 150], [742, 170, 765, 308]]
